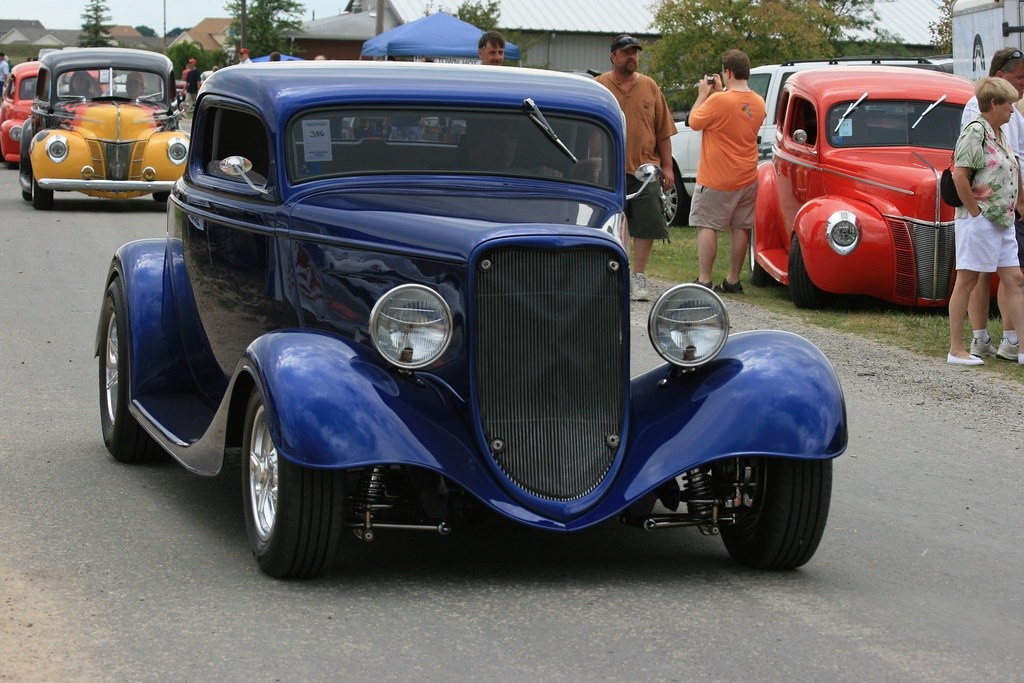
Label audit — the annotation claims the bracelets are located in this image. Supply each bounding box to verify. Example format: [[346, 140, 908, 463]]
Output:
[[87, 76, 92, 81], [425, 121, 430, 128]]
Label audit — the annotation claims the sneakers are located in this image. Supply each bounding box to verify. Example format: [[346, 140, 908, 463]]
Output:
[[631, 273, 650, 302], [996, 338, 1019, 363], [970, 337, 997, 357]]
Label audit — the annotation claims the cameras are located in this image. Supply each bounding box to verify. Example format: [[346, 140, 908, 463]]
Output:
[[706, 76, 715, 85]]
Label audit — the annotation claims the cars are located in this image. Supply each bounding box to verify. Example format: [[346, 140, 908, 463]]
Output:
[[20, 47, 191, 212], [0, 62, 118, 170], [747, 64, 1002, 308], [94, 59, 851, 580]]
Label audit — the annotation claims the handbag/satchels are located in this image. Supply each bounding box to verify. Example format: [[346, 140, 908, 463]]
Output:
[[940, 121, 986, 207]]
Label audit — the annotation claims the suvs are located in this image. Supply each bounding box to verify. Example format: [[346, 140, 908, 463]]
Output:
[[658, 57, 958, 227]]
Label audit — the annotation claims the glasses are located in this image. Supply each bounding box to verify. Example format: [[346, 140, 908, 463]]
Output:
[[240, 53, 244, 55], [995, 51, 1021, 77], [613, 37, 640, 44]]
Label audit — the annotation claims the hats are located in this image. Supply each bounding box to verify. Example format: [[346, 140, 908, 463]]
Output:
[[239, 48, 249, 54], [188, 58, 197, 63], [612, 34, 643, 51]]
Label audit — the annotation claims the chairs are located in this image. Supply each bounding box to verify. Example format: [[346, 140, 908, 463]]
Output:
[[908, 113, 953, 145], [830, 119, 868, 144]]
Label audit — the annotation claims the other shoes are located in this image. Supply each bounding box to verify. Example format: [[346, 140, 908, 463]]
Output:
[[947, 351, 985, 367], [694, 278, 744, 294]]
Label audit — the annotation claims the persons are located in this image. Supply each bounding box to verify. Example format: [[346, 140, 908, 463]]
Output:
[[237, 48, 253, 65], [447, 118, 603, 184], [269, 52, 326, 62], [590, 36, 677, 300], [0, 52, 38, 104], [181, 59, 220, 110], [467, 117, 564, 177], [689, 49, 765, 293], [478, 31, 505, 66], [350, 58, 442, 141], [62, 71, 103, 98], [946, 46, 1024, 366], [115, 72, 156, 102]]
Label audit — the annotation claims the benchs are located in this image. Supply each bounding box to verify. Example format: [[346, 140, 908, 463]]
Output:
[[296, 138, 459, 175]]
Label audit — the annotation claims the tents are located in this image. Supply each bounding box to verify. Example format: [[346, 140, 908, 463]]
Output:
[[250, 54, 304, 62], [359, 12, 521, 67]]
